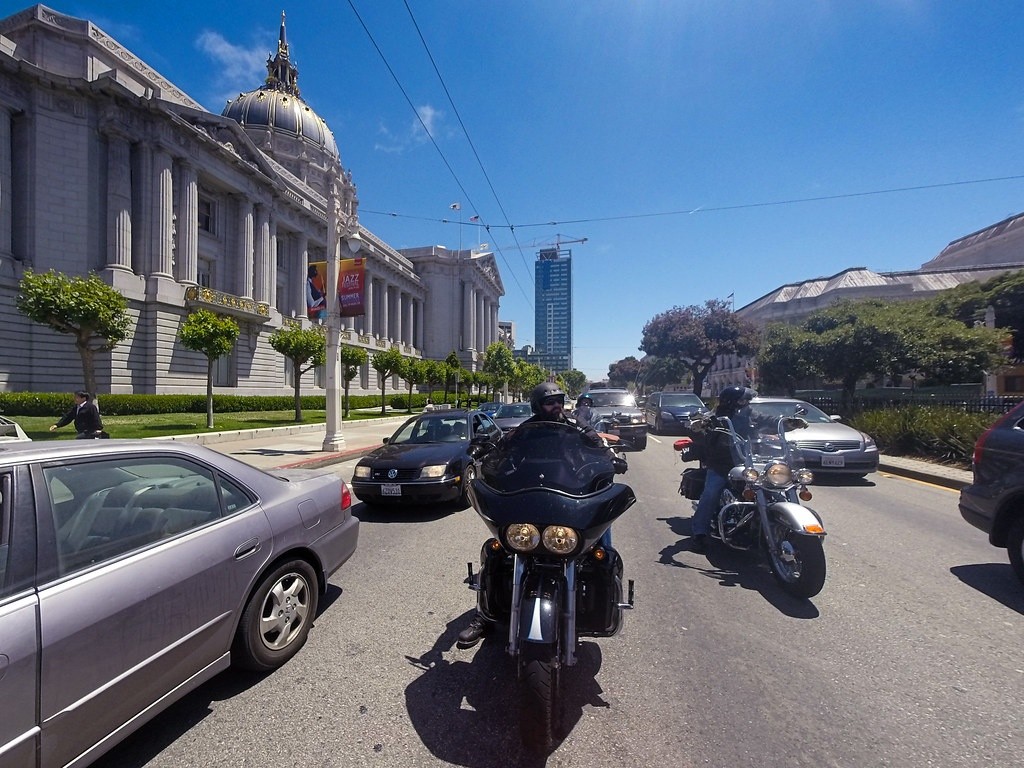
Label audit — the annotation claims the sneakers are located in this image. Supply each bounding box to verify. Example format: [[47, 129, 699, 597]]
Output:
[[458, 618, 493, 648]]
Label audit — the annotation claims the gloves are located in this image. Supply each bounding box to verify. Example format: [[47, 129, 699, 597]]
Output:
[[610, 457, 627, 474], [786, 415, 809, 430], [701, 416, 721, 430]]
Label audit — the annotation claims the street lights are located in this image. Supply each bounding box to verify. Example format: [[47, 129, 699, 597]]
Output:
[[322, 192, 363, 452]]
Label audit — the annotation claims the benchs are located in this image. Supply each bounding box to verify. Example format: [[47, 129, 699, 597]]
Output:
[[60, 483, 229, 565]]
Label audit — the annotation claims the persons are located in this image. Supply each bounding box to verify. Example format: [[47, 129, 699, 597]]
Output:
[[573, 394, 605, 433], [50, 389, 103, 439], [691, 386, 809, 551], [458, 382, 629, 647], [307, 264, 325, 307]]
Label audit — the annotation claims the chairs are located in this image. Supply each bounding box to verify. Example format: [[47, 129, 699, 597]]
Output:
[[422, 422, 465, 440]]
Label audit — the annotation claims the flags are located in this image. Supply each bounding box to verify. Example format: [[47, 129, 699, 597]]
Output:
[[450, 202, 460, 210], [470, 216, 478, 222]]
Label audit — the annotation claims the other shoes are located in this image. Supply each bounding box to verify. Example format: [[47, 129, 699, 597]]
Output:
[[690, 528, 705, 551]]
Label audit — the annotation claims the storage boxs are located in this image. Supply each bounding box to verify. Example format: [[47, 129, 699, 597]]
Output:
[[681, 472, 706, 499]]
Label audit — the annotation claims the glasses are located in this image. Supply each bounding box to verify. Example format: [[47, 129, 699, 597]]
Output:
[[544, 398, 563, 405]]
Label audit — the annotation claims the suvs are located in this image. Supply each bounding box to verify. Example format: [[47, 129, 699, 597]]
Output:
[[563, 387, 648, 451], [958, 400, 1024, 585]]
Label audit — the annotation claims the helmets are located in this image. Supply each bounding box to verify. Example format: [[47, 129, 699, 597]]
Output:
[[576, 393, 594, 408], [530, 382, 565, 413], [719, 386, 758, 409]]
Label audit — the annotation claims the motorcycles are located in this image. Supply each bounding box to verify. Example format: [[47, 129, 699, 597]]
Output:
[[462, 407, 635, 757], [674, 404, 827, 598]]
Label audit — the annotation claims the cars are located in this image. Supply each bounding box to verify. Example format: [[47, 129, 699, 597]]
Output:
[[748, 398, 879, 480], [0, 439, 359, 768], [644, 392, 710, 435], [351, 402, 536, 507]]
